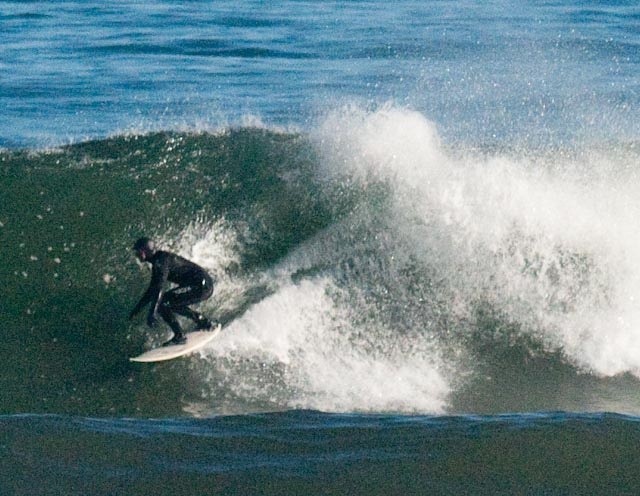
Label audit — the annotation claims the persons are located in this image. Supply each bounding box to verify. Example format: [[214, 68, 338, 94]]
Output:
[[129, 237, 215, 347]]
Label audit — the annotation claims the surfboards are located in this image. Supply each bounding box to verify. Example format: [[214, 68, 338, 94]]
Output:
[[129, 321, 221, 362]]
